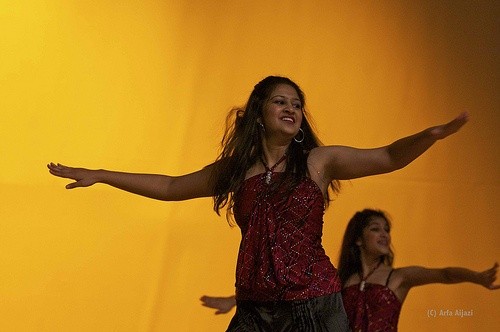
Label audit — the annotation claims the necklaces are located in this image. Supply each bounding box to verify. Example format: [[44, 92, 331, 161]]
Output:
[[259, 148, 289, 184], [355, 260, 381, 292]]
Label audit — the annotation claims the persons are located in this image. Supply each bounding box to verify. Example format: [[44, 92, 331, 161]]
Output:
[[47, 75, 470, 332], [201, 208, 500, 332]]
